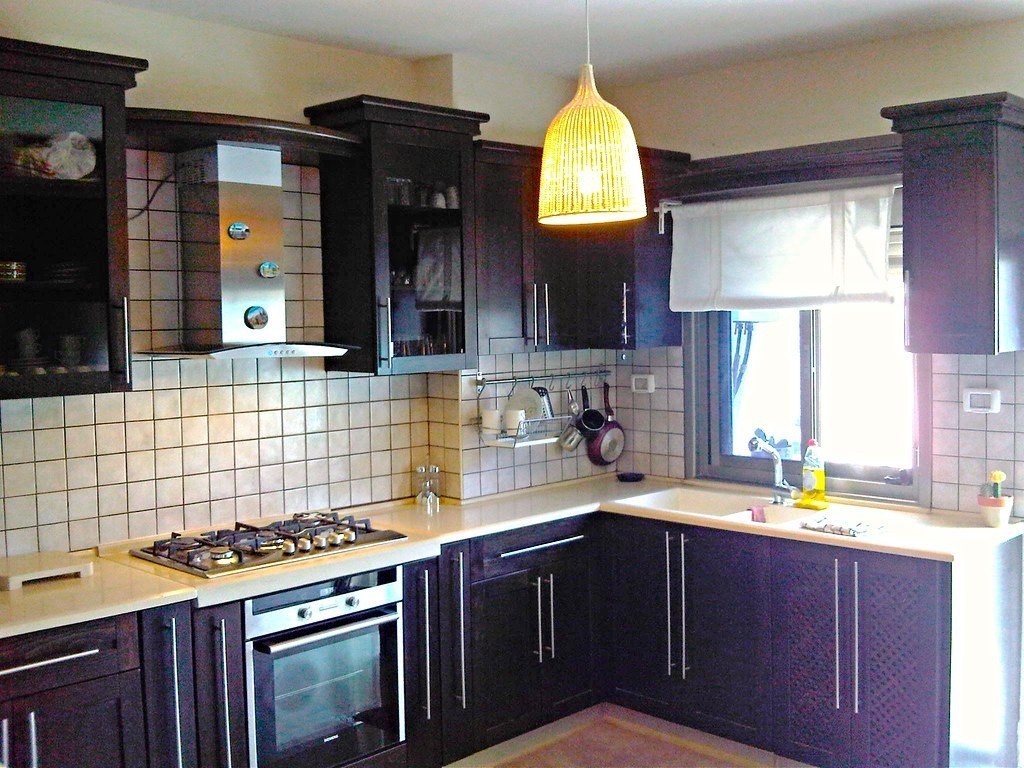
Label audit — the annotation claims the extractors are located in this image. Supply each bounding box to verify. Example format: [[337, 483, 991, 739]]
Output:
[[126, 137, 364, 365]]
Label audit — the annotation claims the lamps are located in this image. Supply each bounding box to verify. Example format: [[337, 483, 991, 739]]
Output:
[[538, 0, 647, 224]]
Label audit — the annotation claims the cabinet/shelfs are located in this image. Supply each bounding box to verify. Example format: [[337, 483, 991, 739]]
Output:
[[0, 36, 149, 401], [478, 139, 590, 356], [600, 510, 775, 768], [0, 556, 197, 768], [438, 511, 606, 768], [770, 537, 1022, 768], [591, 147, 690, 350], [881, 91, 1024, 355], [303, 94, 490, 376]]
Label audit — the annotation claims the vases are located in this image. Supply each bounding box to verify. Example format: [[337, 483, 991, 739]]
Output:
[[977, 493, 1014, 526]]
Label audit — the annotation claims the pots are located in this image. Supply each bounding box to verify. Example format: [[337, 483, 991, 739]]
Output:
[[575, 386, 606, 438], [587, 383, 626, 468]]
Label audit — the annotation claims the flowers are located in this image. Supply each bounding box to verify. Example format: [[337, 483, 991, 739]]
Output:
[[981, 470, 1006, 498]]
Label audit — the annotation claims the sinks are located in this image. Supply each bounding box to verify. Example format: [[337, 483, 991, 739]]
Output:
[[609, 487, 773, 517], [726, 506, 819, 525]]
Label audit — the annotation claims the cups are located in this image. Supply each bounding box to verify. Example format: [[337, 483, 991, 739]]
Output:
[[507, 410, 526, 435], [481, 409, 501, 435], [13, 328, 42, 360], [57, 335, 80, 364], [558, 425, 583, 452]]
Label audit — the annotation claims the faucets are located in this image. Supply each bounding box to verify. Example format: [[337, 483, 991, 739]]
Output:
[[748, 436, 783, 504]]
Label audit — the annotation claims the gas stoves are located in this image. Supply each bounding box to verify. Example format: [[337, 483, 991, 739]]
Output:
[[129, 507, 411, 579]]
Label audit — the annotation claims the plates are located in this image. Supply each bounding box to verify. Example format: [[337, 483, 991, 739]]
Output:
[[7, 357, 50, 370], [506, 387, 544, 434]]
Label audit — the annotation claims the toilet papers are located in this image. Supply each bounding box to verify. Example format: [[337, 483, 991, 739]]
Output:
[[481, 409, 501, 434], [505, 409, 526, 436]]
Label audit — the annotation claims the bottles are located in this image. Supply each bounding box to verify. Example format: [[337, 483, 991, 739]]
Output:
[[801, 439, 826, 500], [385, 176, 462, 210]]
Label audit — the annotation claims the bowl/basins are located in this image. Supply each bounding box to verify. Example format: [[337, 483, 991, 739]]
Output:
[[0, 260, 26, 283]]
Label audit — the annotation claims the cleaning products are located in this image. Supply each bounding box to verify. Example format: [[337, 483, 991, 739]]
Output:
[[802, 438, 826, 502]]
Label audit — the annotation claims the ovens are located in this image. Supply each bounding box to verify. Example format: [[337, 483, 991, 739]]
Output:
[[237, 567, 411, 768]]
